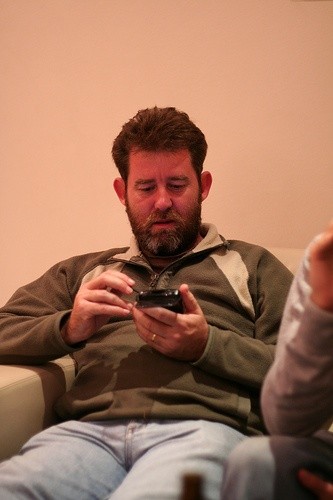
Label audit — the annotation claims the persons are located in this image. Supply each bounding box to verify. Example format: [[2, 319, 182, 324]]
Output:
[[224, 226, 331, 500], [0, 107, 295, 500]]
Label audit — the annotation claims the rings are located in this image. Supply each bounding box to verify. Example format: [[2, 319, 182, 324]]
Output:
[[152, 334, 156, 341]]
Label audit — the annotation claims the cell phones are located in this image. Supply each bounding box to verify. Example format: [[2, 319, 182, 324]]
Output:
[[136, 289, 184, 314]]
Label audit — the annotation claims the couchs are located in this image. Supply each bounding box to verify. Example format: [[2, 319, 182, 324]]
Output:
[[0, 245, 302, 462]]
